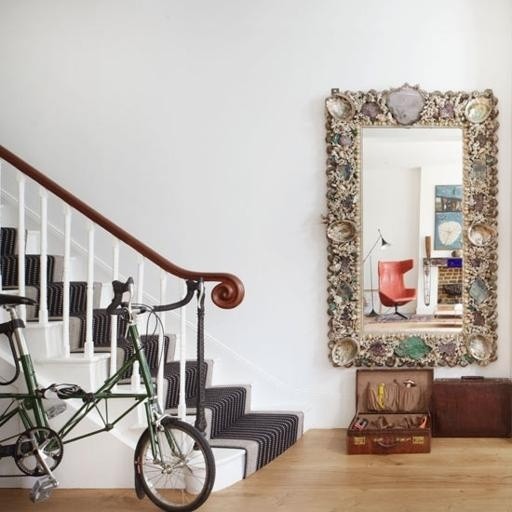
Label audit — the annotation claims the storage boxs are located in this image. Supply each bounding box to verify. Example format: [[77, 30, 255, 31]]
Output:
[[434, 375, 511, 437]]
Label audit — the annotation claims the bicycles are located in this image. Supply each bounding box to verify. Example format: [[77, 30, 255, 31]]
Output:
[[0, 277, 216, 512]]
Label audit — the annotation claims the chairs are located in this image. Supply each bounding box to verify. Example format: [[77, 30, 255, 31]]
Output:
[[376, 257, 417, 320]]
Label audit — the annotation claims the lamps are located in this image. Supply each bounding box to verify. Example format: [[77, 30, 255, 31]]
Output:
[[363, 227, 391, 318]]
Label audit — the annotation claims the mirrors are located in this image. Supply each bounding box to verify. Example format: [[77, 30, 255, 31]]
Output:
[[324, 87, 503, 369]]
